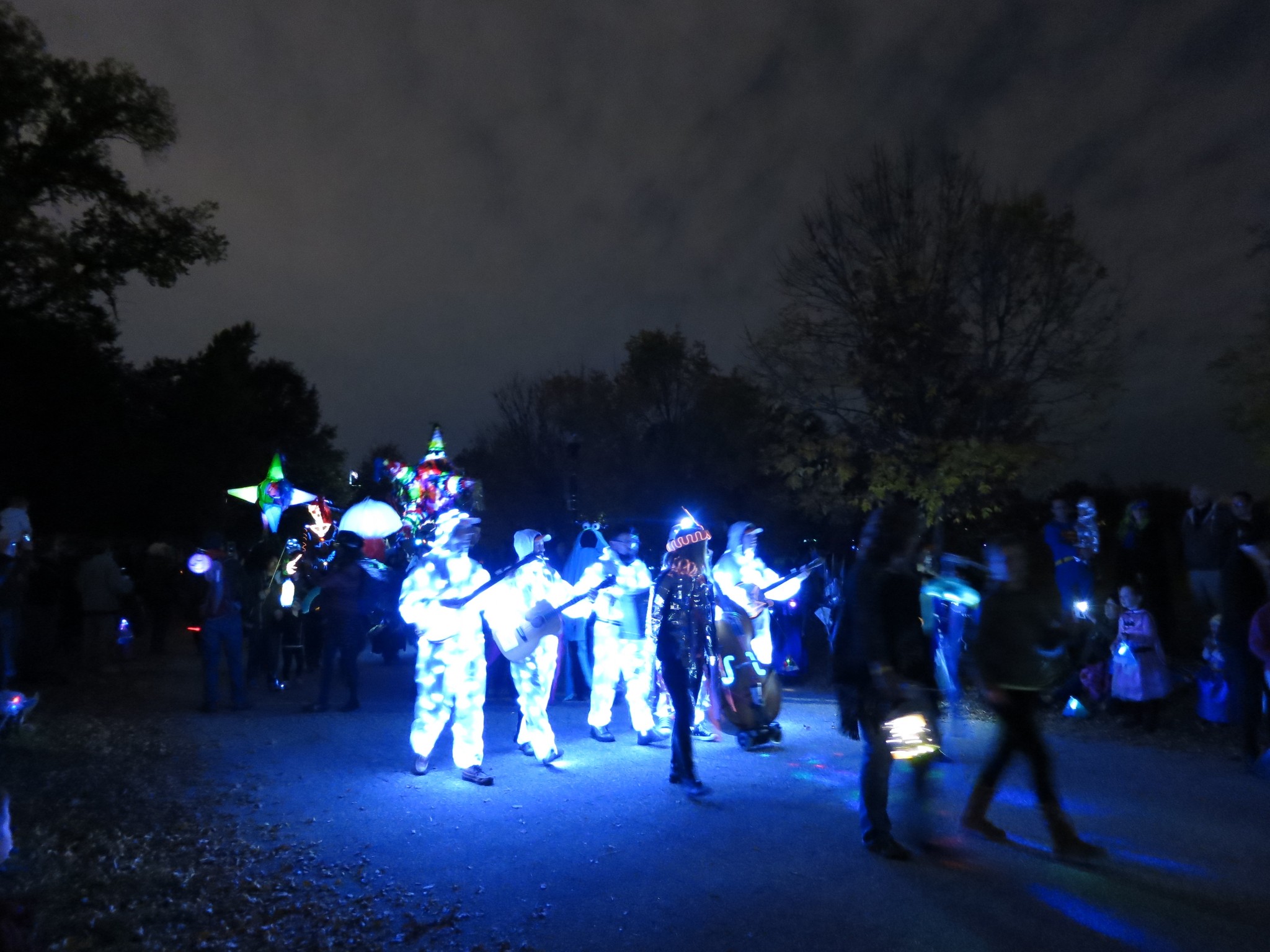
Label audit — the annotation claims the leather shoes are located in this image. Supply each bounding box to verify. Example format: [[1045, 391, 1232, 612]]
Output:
[[516, 743, 535, 755], [638, 729, 670, 745], [413, 754, 429, 775], [591, 725, 614, 743], [462, 766, 494, 786], [542, 750, 563, 765]]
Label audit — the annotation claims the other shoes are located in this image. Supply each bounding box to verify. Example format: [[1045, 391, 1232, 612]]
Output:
[[1054, 840, 1108, 862], [668, 774, 684, 781], [960, 812, 1004, 842], [867, 839, 910, 862], [677, 773, 702, 786]]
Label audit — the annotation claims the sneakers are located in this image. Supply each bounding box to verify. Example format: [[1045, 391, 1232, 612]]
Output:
[[691, 725, 715, 739]]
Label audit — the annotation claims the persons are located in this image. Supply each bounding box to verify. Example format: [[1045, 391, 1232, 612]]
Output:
[[815, 479, 1270, 735], [0, 776, 33, 915], [832, 502, 943, 860], [958, 535, 1107, 864], [708, 521, 811, 749], [484, 523, 667, 766], [399, 509, 491, 786], [0, 490, 414, 725], [652, 508, 754, 798], [1240, 603, 1270, 774]]
